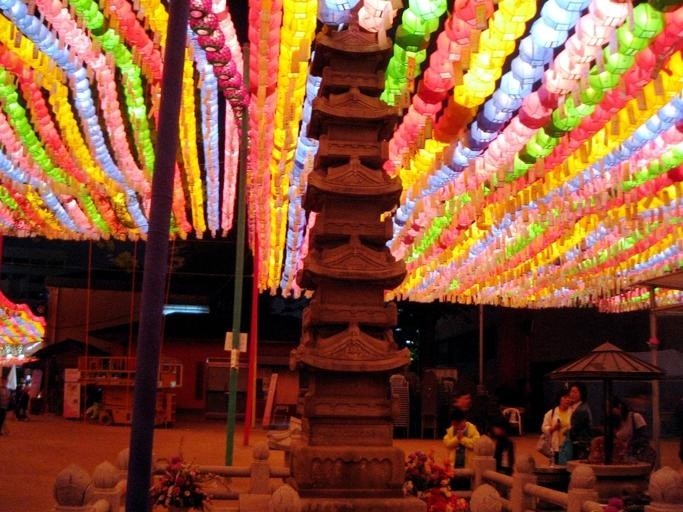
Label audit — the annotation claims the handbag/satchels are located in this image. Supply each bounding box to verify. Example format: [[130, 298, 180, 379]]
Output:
[[537, 433, 553, 458]]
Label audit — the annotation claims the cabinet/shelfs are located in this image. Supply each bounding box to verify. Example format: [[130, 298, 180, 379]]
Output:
[[77, 357, 183, 426]]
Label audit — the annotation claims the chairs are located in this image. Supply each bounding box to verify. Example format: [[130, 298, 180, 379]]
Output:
[[388, 374, 411, 440], [501, 408, 522, 435]]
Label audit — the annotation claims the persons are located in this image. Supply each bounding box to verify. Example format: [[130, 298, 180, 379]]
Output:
[[442, 388, 515, 492], [0, 376, 34, 433], [536, 382, 656, 469]]
